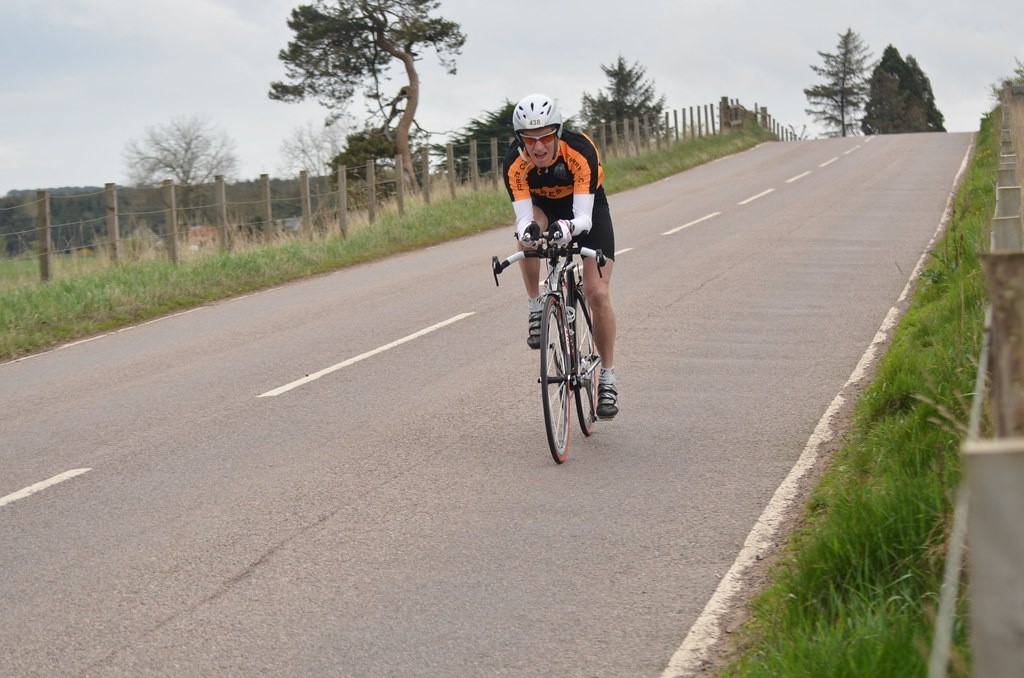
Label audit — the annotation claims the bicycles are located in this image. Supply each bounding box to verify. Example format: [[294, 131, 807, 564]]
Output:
[[491, 230, 614, 465]]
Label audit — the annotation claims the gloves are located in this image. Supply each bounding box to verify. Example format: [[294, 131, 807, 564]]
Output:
[[546, 219, 575, 249], [517, 220, 541, 249]]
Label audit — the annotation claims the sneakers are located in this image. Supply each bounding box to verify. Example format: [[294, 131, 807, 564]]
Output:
[[597, 381, 619, 418], [526, 309, 549, 349]]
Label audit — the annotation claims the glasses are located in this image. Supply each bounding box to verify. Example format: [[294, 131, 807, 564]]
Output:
[[520, 127, 558, 145]]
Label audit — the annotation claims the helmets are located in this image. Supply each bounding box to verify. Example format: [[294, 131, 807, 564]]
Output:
[[512, 93, 563, 143]]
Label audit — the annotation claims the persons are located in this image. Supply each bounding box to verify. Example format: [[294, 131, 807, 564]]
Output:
[[502, 94, 618, 419]]
